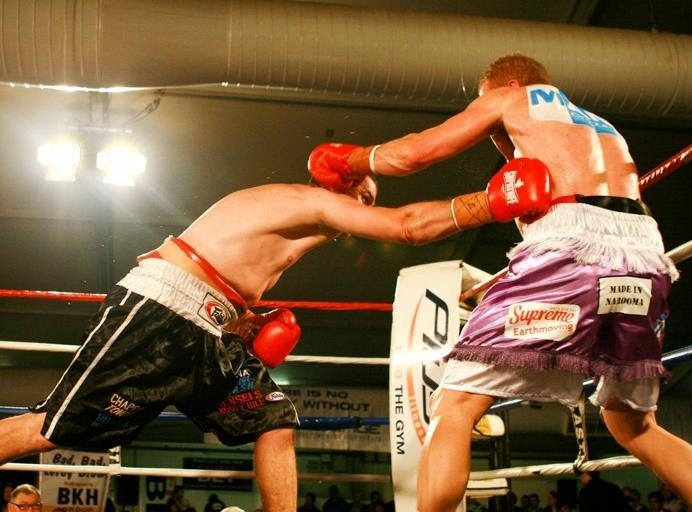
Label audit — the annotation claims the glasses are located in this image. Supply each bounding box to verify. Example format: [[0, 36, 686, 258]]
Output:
[[7, 500, 42, 511]]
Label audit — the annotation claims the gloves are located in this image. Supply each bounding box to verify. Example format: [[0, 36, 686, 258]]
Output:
[[228, 307, 300, 366], [452, 158, 555, 232], [307, 140, 381, 194]]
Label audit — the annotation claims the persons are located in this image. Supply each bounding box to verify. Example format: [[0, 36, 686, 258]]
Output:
[[1, 468, 688, 512], [0, 159, 555, 512], [307, 54, 692, 512]]
